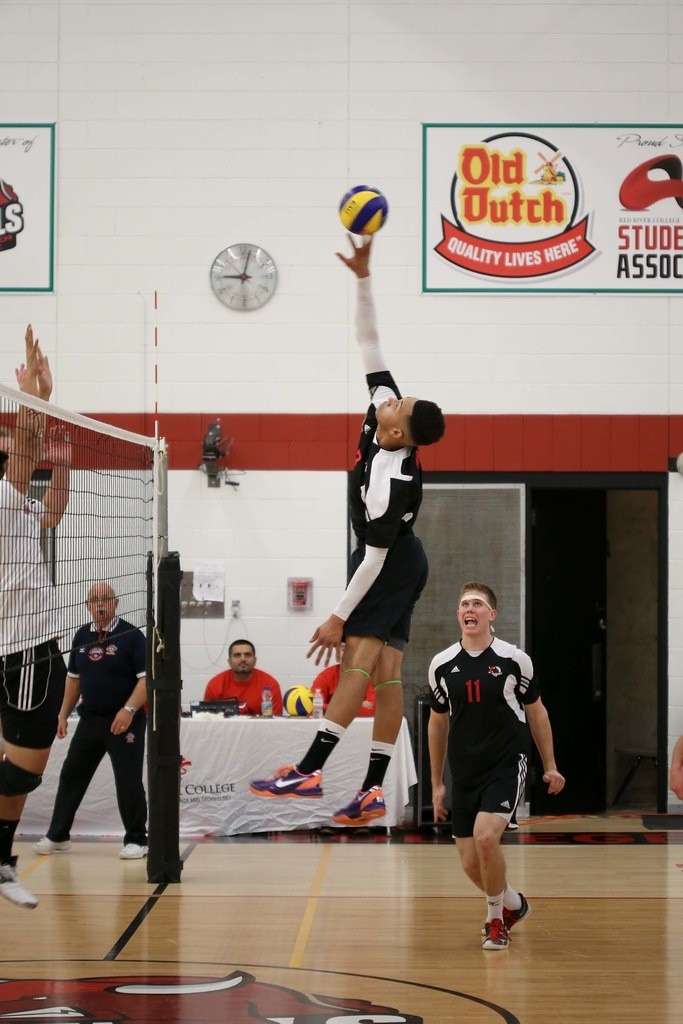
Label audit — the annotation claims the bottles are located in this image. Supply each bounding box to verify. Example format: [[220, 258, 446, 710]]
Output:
[[313, 689, 323, 719], [261, 687, 272, 718]]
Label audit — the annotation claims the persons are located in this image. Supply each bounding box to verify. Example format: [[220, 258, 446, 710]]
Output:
[[310, 638, 377, 719], [247, 231, 447, 827], [430, 584, 566, 950], [0, 324, 70, 908], [33, 580, 148, 861], [204, 640, 284, 718]]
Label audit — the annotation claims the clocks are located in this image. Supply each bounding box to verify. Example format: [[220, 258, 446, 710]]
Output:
[[211, 243, 278, 312]]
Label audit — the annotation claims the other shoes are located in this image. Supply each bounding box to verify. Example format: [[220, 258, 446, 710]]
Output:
[[0, 861, 42, 909]]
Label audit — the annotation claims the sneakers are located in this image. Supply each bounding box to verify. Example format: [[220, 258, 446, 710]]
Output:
[[330, 784, 388, 827], [120, 842, 150, 860], [481, 919, 513, 949], [31, 836, 71, 855], [247, 764, 328, 800], [498, 893, 533, 934]]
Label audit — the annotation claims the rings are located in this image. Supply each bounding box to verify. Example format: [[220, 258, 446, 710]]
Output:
[[121, 726, 125, 729]]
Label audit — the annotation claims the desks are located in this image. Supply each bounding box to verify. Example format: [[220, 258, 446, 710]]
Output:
[[2, 717, 419, 836]]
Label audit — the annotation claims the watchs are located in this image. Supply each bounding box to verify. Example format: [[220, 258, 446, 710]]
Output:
[[124, 705, 136, 715]]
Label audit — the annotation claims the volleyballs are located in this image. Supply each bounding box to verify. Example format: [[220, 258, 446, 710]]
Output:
[[339, 184, 388, 236], [282, 685, 314, 716]]
